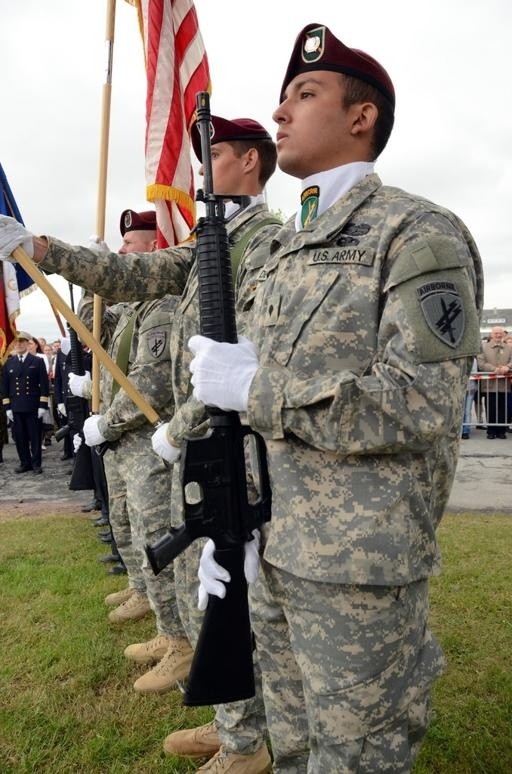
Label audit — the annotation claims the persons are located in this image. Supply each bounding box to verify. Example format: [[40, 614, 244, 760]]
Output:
[[462, 326, 512, 440], [53, 211, 195, 695], [0, 330, 74, 474], [0, 119, 283, 774], [196, 23, 482, 774]]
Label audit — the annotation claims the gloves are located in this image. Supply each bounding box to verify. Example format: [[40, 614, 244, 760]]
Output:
[[38, 408, 45, 418], [0, 214, 33, 263], [197, 529, 261, 611], [58, 403, 66, 417], [6, 410, 14, 423], [151, 423, 181, 463], [73, 433, 81, 453], [83, 415, 106, 447], [187, 334, 261, 412], [69, 370, 91, 399]]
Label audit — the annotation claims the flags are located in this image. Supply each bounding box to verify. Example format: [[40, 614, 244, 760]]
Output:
[[0, 259, 21, 364], [1, 169, 43, 299], [124, 0, 213, 251]]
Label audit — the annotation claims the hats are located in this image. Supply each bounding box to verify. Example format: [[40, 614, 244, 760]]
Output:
[[192, 116, 274, 175], [120, 209, 159, 233], [16, 334, 35, 347], [280, 23, 395, 125]]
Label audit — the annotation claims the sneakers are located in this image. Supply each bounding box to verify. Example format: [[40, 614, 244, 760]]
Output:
[[164, 719, 272, 774], [124, 633, 194, 696], [42, 438, 51, 450], [65, 481, 71, 486], [98, 555, 127, 576], [105, 585, 151, 624]]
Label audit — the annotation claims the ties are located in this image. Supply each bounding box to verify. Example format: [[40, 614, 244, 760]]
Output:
[[20, 355, 23, 363]]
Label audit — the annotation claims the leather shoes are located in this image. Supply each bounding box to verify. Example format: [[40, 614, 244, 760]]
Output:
[[64, 471, 72, 475], [15, 466, 26, 473], [82, 502, 113, 543], [61, 454, 72, 461], [34, 466, 42, 474]]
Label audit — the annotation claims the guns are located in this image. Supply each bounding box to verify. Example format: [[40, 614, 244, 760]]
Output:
[[143, 93, 273, 707], [56, 280, 96, 490]]
